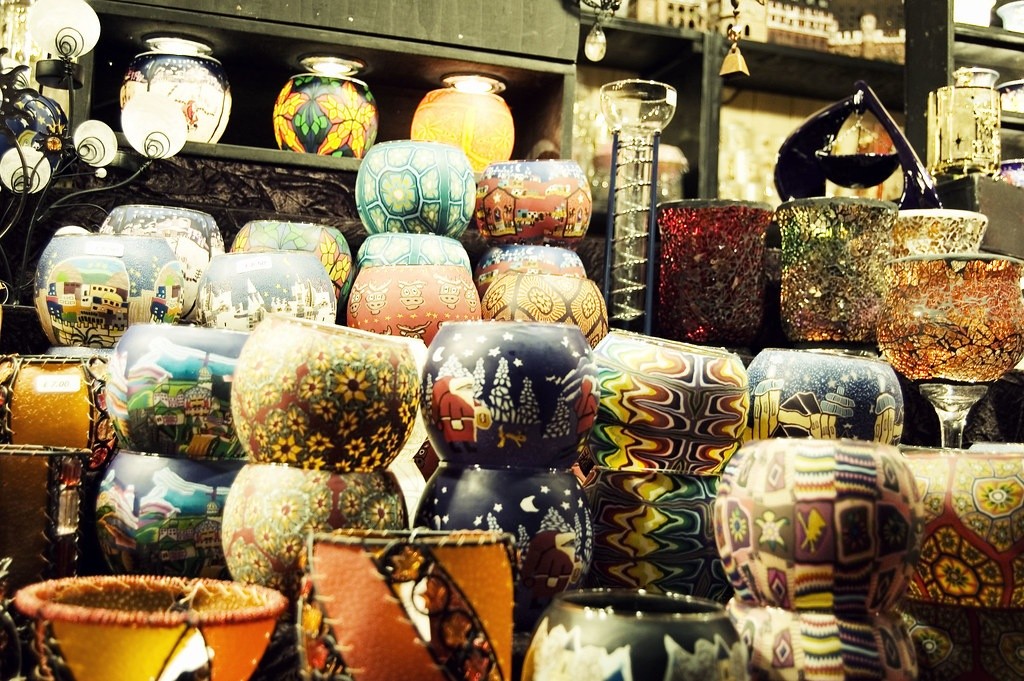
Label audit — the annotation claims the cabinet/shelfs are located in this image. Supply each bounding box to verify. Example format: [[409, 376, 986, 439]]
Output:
[[61, 0, 1024, 225]]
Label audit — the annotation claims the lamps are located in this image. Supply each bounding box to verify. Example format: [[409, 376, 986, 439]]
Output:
[[0, 0, 189, 354]]
